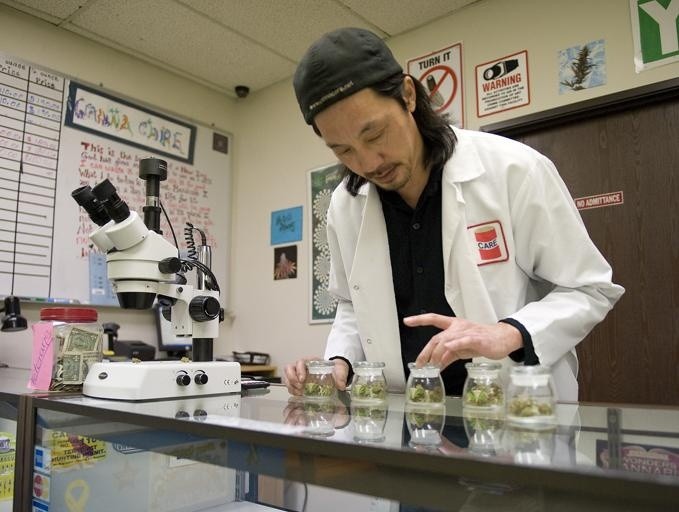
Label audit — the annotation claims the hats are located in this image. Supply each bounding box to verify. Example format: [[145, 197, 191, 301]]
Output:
[[291, 25, 404, 125]]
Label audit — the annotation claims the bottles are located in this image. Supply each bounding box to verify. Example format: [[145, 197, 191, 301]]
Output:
[[301, 405, 555, 469], [299, 356, 559, 429], [32, 304, 105, 389]]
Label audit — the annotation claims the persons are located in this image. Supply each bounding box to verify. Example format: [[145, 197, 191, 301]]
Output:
[[280, 396, 578, 509], [280, 27, 629, 404]]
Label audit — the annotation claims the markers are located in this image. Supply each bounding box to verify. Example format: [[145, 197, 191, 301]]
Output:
[[16, 296, 81, 306]]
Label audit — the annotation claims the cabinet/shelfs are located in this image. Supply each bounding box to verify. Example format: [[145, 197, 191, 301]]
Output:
[[1, 381, 679, 510]]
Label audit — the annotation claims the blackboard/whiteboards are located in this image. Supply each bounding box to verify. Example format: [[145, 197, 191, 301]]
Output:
[[0, 50, 235, 320]]
